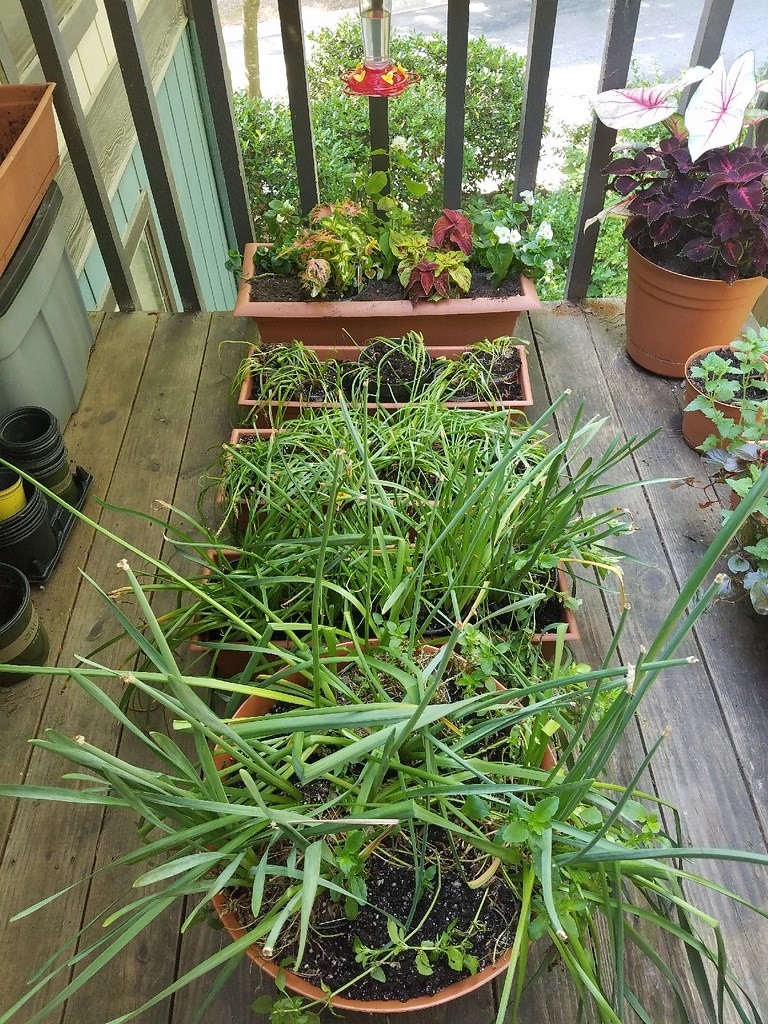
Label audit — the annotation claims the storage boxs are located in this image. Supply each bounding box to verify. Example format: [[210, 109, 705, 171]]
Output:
[[0, 81, 61, 274]]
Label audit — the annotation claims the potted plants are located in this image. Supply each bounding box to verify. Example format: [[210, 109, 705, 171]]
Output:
[[0, 339, 768, 1024]]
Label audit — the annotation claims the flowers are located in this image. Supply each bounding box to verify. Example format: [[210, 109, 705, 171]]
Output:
[[570, 51, 768, 282], [221, 151, 559, 301]]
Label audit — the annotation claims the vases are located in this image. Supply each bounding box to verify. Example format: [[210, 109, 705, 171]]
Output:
[[625, 216, 768, 370], [237, 241, 543, 344]]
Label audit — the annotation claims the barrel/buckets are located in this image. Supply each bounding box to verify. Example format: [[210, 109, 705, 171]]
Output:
[[0, 406, 77, 568], [0, 562, 49, 684]]
